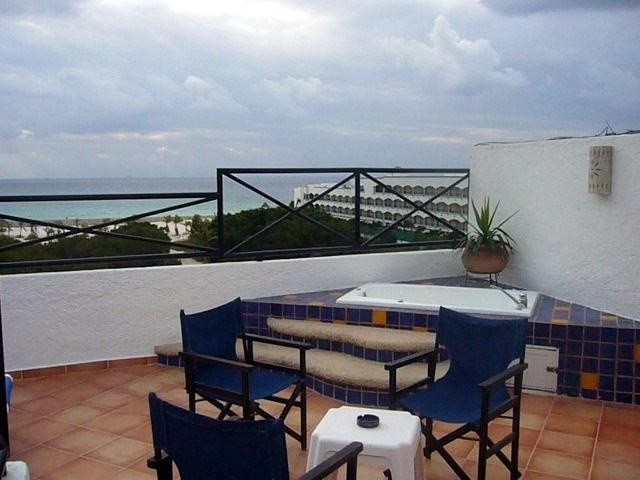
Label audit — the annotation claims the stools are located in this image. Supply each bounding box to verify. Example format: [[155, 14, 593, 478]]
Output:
[[305, 404, 424, 480]]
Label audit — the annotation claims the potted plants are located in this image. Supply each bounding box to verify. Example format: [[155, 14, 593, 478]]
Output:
[[457, 197, 522, 286]]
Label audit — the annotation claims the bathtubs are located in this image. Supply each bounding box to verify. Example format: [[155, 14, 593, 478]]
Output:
[[336, 282, 538, 317]]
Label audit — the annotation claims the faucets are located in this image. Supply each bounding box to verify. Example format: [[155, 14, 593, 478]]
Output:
[[490, 284, 518, 305]]
